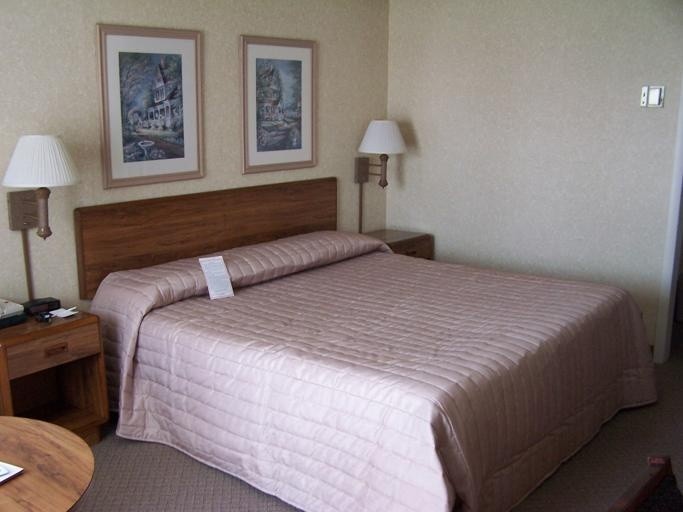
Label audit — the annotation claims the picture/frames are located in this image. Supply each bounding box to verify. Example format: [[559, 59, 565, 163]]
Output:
[[238, 32, 320, 173], [97, 22, 208, 188]]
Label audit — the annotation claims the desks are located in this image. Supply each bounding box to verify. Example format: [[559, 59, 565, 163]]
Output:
[[0, 413, 90, 511]]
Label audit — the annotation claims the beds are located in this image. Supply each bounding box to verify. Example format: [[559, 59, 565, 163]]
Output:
[[72, 178, 656, 509]]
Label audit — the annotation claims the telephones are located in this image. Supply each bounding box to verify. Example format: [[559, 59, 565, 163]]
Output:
[[0, 299, 24, 320]]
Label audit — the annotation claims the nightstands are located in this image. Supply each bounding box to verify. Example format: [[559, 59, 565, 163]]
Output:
[[361, 228, 432, 261], [0, 308, 109, 446]]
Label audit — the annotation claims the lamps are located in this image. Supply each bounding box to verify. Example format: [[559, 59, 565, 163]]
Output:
[[0, 131, 83, 299], [352, 120, 406, 233]]
